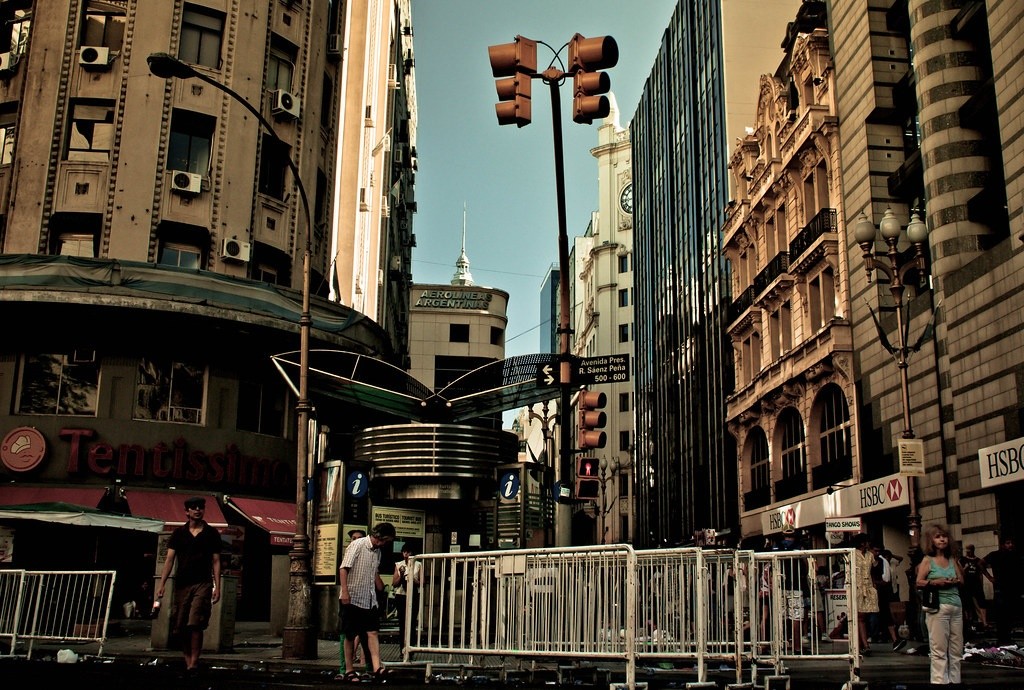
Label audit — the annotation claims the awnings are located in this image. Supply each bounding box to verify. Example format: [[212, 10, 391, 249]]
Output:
[[0, 486, 228, 534], [229, 497, 298, 547]]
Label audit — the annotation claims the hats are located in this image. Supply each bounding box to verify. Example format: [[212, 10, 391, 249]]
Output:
[[401, 543, 417, 554], [184, 497, 206, 509], [371, 523, 395, 542]]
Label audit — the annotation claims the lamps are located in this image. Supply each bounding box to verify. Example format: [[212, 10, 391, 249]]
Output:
[[827, 484, 850, 495]]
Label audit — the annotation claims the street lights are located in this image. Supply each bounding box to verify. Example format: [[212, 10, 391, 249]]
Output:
[[848, 202, 943, 640], [598, 454, 619, 545], [527, 397, 559, 551], [141, 51, 321, 664]]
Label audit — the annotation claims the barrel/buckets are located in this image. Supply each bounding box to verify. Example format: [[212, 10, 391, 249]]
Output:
[[123, 603, 136, 618]]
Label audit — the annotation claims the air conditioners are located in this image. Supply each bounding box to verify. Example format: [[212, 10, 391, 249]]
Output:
[[392, 256, 400, 270], [220, 238, 251, 264], [389, 64, 397, 86], [171, 170, 202, 194], [0, 52, 16, 72], [271, 89, 300, 119], [79, 46, 109, 65], [394, 149, 403, 163], [73, 349, 95, 363], [330, 33, 343, 57]]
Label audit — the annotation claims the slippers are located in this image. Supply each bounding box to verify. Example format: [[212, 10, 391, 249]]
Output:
[[334, 668, 396, 682]]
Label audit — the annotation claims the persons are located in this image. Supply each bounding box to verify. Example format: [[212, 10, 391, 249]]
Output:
[[334, 523, 396, 682], [759, 525, 907, 655], [958, 537, 1024, 627], [393, 547, 425, 660], [156, 497, 224, 671], [917, 522, 964, 690]]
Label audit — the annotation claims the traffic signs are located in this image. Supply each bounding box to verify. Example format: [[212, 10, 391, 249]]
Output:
[[535, 353, 632, 388]]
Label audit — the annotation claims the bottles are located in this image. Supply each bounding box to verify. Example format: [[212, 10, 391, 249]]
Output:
[[149, 596, 162, 619]]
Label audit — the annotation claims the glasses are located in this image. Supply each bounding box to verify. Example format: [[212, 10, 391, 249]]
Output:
[[187, 503, 205, 510]]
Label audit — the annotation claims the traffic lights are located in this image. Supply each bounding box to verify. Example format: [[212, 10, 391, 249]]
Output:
[[575, 457, 601, 502], [488, 35, 534, 128], [568, 32, 620, 125], [554, 482, 575, 506], [555, 397, 562, 425], [576, 389, 609, 452]]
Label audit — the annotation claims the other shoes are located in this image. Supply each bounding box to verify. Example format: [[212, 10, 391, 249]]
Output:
[[821, 634, 833, 643], [802, 637, 810, 644], [861, 649, 872, 656], [893, 640, 906, 653]]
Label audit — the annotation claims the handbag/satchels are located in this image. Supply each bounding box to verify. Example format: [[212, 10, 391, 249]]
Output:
[[922, 586, 939, 613]]
